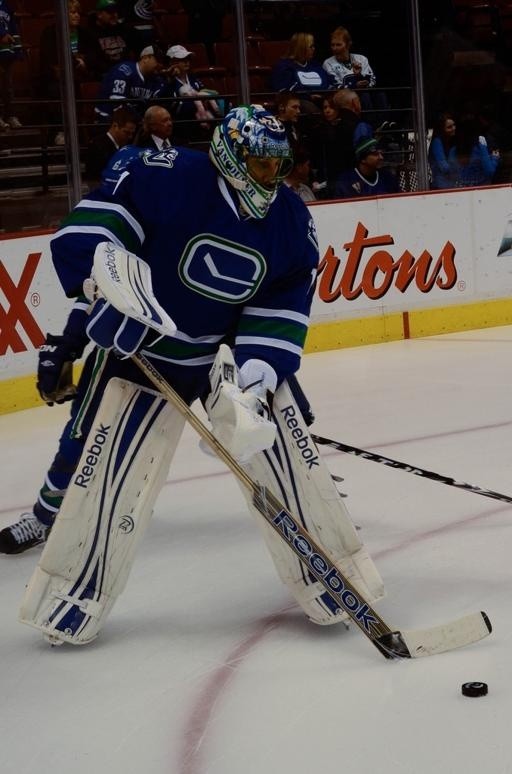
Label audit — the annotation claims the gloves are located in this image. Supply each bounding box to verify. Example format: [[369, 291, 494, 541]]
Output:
[[37, 332, 80, 407]]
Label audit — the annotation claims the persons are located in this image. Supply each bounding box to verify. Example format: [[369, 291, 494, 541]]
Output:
[[25, 1, 219, 190], [262, 26, 399, 201], [182, 2, 224, 65], [17, 102, 390, 647], [430, 111, 505, 190], [2, 1, 22, 128]]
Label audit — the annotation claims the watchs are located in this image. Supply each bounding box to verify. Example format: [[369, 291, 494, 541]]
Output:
[[0, 146, 313, 553]]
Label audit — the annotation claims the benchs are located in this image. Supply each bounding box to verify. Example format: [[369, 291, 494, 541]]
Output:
[[0, 1, 332, 214]]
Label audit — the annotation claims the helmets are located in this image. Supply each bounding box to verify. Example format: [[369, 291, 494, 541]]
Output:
[[208, 102, 293, 219]]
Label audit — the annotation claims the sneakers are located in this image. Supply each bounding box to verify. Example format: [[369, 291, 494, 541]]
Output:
[[0, 513, 51, 553], [0, 117, 21, 131]]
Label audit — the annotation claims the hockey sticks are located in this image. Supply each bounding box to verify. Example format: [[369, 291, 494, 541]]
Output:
[[133, 351, 492, 659]]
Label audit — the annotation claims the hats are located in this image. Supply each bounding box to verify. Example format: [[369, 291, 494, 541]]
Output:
[[354, 135, 381, 158], [95, 0, 118, 9], [138, 44, 193, 64]]
